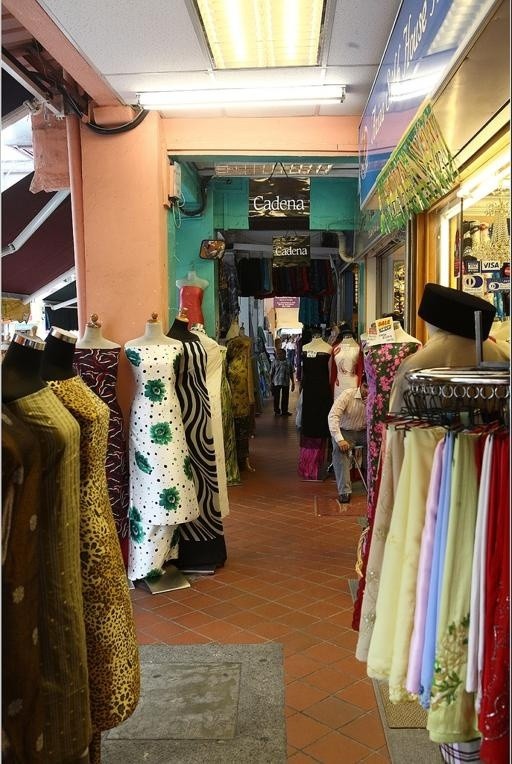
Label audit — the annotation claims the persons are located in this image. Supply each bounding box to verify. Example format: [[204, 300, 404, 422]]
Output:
[[44, 325, 139, 762], [125, 318, 198, 569], [333, 334, 361, 402], [363, 320, 423, 519], [281, 338, 287, 350], [167, 317, 229, 568], [190, 321, 241, 519], [174, 269, 209, 331], [73, 322, 128, 570], [328, 375, 369, 503], [1, 329, 88, 764], [301, 335, 332, 481], [269, 349, 295, 416], [286, 338, 297, 368]]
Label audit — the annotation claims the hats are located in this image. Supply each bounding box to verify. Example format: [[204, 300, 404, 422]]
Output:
[[418, 283, 497, 342]]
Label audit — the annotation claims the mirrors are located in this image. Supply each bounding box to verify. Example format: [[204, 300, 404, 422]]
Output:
[[198, 239, 224, 260]]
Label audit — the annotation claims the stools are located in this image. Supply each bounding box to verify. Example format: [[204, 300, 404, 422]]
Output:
[[323, 445, 367, 495]]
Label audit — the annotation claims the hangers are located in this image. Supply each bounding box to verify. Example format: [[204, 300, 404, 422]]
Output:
[[375, 384, 510, 440]]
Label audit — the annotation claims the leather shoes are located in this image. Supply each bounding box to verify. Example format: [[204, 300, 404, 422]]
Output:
[[340, 492, 350, 504]]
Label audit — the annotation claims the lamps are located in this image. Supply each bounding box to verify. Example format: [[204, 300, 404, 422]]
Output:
[[180, 0, 342, 75]]
